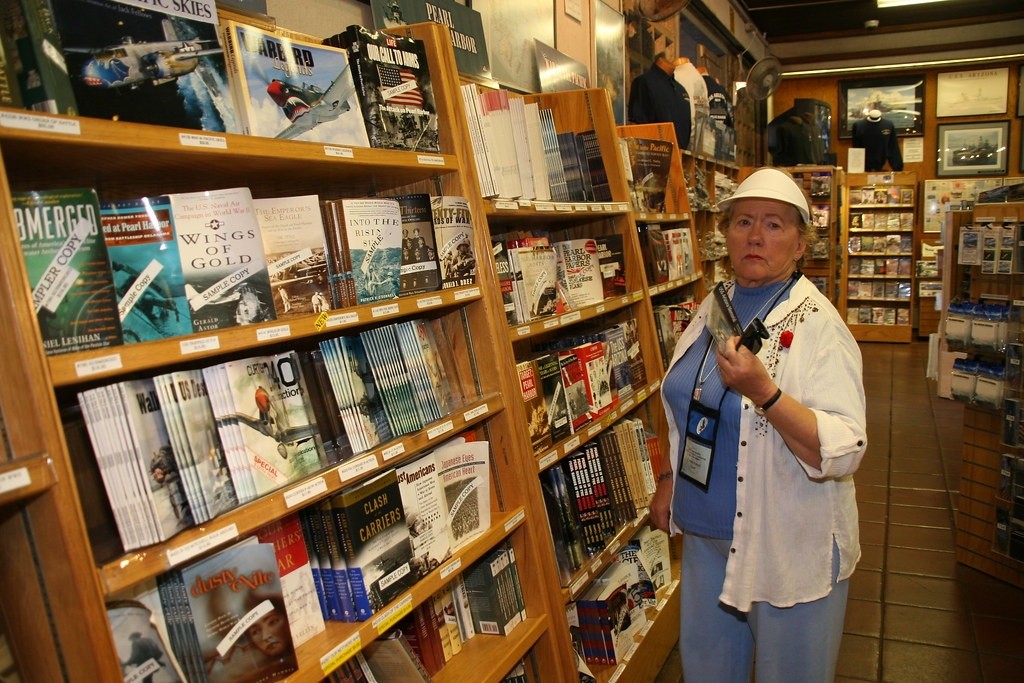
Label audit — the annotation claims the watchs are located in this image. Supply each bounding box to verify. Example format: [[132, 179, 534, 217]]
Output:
[[754, 388, 782, 416]]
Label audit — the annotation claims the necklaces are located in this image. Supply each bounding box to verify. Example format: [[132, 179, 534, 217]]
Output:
[[694, 279, 790, 401]]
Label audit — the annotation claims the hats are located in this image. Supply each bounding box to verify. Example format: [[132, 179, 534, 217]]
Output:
[[717, 170, 810, 227]]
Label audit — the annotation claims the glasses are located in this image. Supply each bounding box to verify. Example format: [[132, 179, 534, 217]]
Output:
[[735, 319, 769, 356]]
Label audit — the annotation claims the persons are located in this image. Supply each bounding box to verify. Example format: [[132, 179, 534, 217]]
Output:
[[402, 229, 435, 287], [247, 595, 293, 663], [444, 235, 475, 277], [853, 111, 903, 171], [777, 112, 825, 165], [628, 55, 737, 159], [660, 169, 868, 683]]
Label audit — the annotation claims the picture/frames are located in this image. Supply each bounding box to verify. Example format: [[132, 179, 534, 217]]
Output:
[[936, 120, 1011, 179], [837, 73, 925, 139]]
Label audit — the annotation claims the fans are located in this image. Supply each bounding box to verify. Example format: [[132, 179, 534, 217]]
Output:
[[736, 53, 783, 100], [637, 1, 689, 23]]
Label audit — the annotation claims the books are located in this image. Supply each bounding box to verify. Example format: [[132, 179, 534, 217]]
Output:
[[540, 417, 660, 586], [492, 234, 626, 326], [514, 319, 648, 457], [620, 135, 705, 370], [846, 187, 912, 325], [106, 431, 492, 683], [430, 196, 478, 290], [463, 82, 614, 203], [76, 316, 457, 552], [318, 535, 529, 683], [0, 1, 440, 153], [384, 193, 444, 296], [12, 186, 403, 358], [568, 526, 672, 683]]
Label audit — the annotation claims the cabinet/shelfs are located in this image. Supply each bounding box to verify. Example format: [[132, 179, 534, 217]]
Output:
[[0, 0, 1024, 683]]
